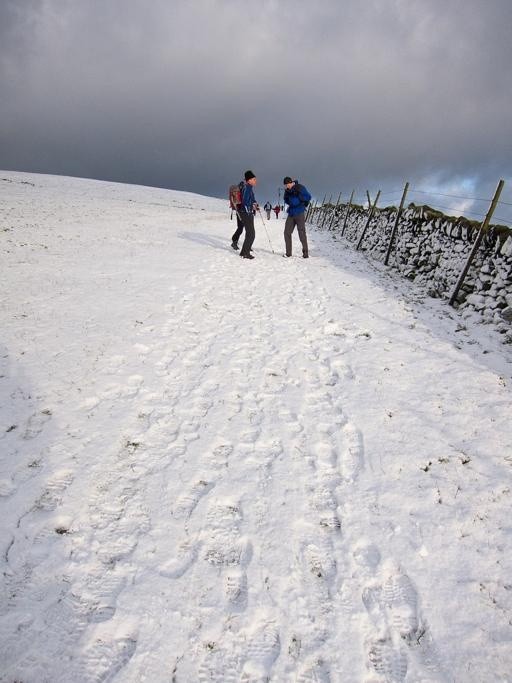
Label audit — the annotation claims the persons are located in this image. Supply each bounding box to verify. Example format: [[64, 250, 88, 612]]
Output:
[[263, 201, 272, 220], [229, 168, 259, 260], [272, 203, 280, 218], [280, 175, 313, 258]]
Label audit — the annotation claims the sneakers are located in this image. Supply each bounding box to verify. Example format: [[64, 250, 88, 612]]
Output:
[[232, 243, 255, 260]]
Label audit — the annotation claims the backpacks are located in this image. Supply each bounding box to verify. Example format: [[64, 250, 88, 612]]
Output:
[[228, 183, 250, 211]]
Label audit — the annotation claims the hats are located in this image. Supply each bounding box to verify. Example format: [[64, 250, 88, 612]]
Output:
[[244, 171, 256, 180], [283, 176, 292, 186]]
